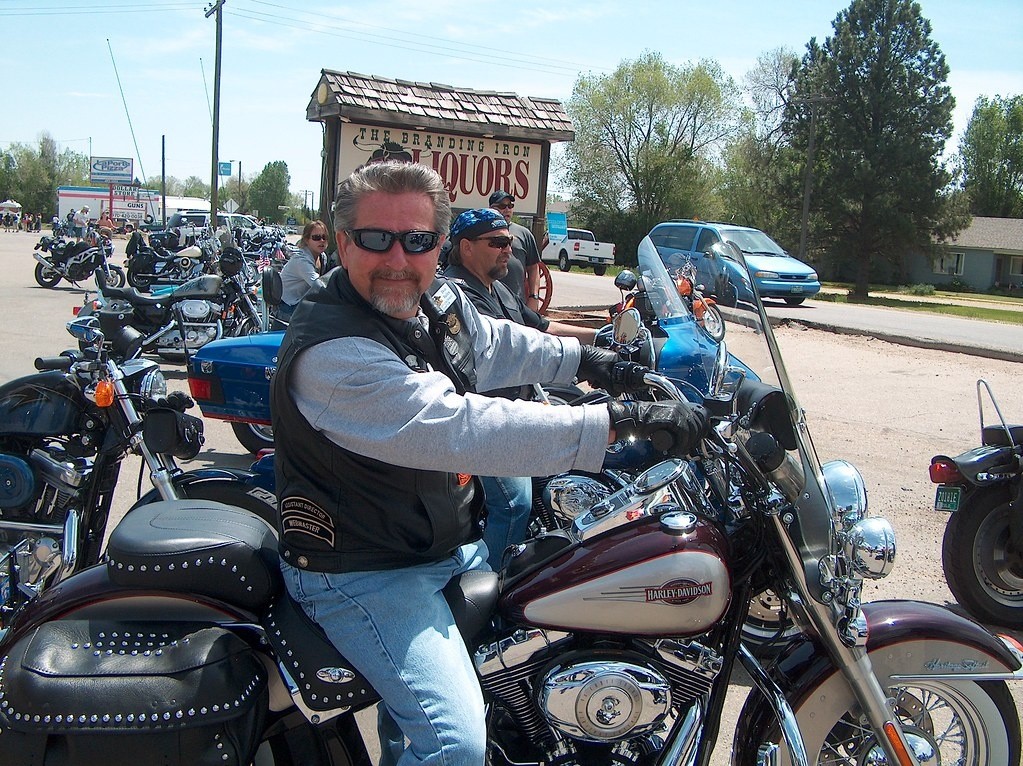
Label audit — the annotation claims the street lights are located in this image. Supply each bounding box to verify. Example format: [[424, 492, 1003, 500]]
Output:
[[204, 0, 228, 232], [300, 189, 314, 224], [230, 159, 241, 208]]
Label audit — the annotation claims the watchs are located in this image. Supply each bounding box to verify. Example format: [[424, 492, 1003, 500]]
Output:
[[528, 294, 539, 300]]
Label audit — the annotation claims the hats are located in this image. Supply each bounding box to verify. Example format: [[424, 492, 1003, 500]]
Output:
[[489, 190, 515, 205], [83, 205, 91, 211], [449, 209, 509, 245]]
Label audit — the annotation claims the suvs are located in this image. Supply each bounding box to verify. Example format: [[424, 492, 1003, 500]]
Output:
[[637, 219, 821, 306], [164, 211, 286, 238]]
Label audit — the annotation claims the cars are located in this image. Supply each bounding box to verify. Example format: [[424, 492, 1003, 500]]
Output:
[[112, 217, 134, 233]]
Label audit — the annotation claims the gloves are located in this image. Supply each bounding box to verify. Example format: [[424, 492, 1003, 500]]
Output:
[[577, 345, 624, 398], [607, 399, 711, 455]]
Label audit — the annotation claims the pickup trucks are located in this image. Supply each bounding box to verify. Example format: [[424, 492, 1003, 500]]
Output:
[[541, 227, 616, 276], [285, 226, 297, 234]]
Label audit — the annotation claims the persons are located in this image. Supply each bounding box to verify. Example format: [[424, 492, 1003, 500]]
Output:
[[441, 206, 604, 577], [268, 161, 712, 766], [490, 190, 541, 314], [277, 220, 330, 317], [414, 234, 425, 243], [382, 232, 386, 241], [67, 205, 117, 244], [2, 213, 41, 233], [52, 214, 59, 237]]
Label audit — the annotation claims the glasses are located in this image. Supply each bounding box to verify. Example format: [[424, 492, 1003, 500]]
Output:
[[492, 204, 514, 209], [309, 234, 328, 240], [472, 235, 513, 249], [344, 228, 440, 254]]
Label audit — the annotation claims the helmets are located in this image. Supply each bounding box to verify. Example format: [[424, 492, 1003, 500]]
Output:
[[219, 247, 243, 276]]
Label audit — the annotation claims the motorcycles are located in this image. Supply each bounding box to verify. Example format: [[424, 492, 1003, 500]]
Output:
[[0, 218, 1023, 766], [33, 225, 126, 291], [591, 253, 726, 350], [930, 380, 1023, 634]]
